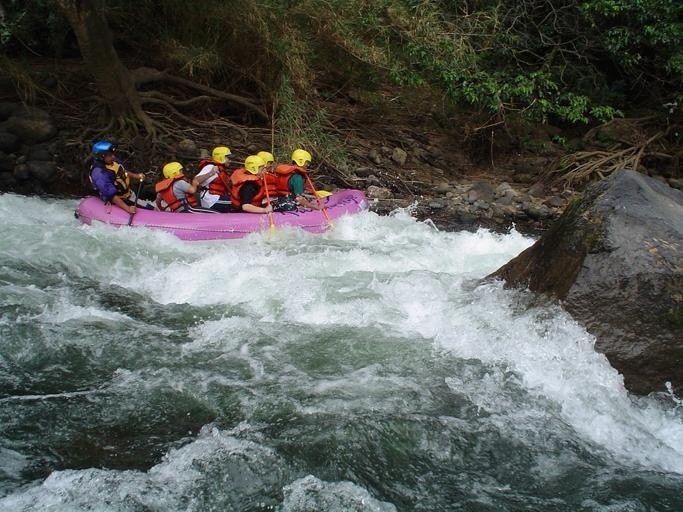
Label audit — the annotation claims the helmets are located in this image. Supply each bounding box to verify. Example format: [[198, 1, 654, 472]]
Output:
[[292, 149, 312, 167], [163, 162, 184, 179], [245, 155, 265, 174], [212, 146, 232, 165], [92, 140, 113, 155], [257, 151, 274, 167]]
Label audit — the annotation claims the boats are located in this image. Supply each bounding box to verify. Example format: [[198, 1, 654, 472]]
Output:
[[73, 189, 367, 240]]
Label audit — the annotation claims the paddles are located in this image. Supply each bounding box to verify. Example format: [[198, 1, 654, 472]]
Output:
[[262, 171, 277, 237]]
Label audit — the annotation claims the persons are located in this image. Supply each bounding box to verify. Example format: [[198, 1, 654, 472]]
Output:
[[253, 152, 279, 202], [89, 141, 155, 214], [156, 161, 200, 213], [275, 148, 325, 210], [229, 155, 274, 214], [193, 146, 239, 213]]
